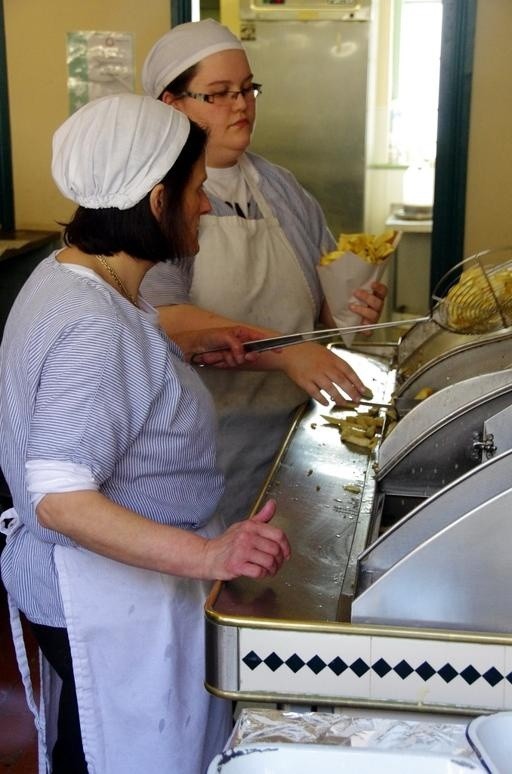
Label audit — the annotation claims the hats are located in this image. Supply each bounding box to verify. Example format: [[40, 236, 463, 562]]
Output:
[[51, 95, 191, 211], [142, 18, 244, 99]]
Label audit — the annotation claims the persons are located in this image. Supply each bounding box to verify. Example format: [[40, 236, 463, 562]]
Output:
[[128, 19, 388, 532], [2, 93, 294, 773]]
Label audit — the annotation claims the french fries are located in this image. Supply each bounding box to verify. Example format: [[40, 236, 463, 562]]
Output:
[[320, 229, 396, 267], [320, 406, 398, 447]]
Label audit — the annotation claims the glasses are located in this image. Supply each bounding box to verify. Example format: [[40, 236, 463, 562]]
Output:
[[186, 82, 262, 105]]
